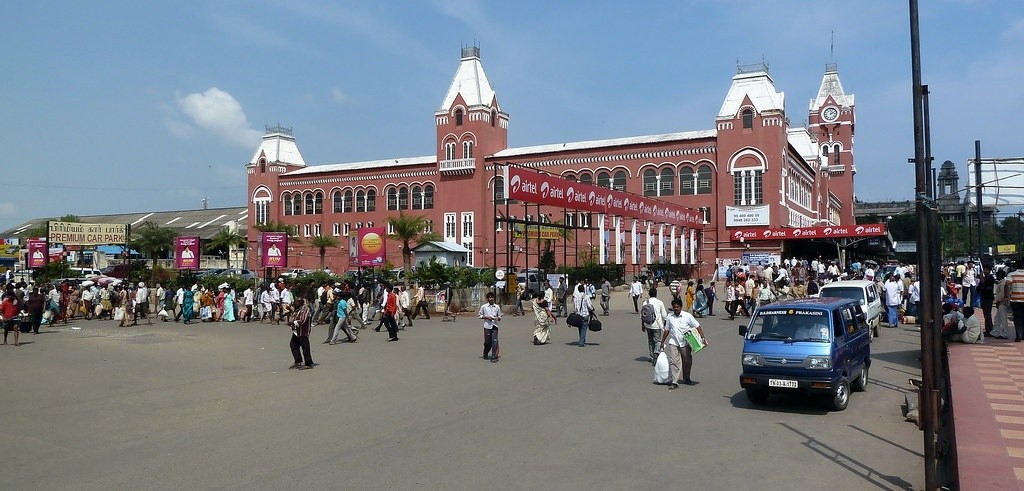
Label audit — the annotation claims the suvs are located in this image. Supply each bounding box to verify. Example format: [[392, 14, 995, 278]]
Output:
[[280, 268, 305, 280]]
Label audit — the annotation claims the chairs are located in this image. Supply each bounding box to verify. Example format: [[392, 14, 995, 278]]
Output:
[[773, 321, 799, 338]]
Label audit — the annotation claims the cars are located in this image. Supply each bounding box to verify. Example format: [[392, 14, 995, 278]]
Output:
[[517, 273, 545, 300], [342, 270, 363, 277], [216, 268, 256, 284], [5, 244, 20, 255], [200, 268, 227, 279], [469, 267, 491, 280]]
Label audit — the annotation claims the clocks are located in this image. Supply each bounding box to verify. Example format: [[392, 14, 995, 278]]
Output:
[[824, 107, 837, 120]]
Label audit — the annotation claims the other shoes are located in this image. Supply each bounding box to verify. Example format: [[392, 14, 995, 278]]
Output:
[[668, 384, 678, 389], [693, 309, 751, 320], [299, 365, 312, 370], [653, 354, 658, 366], [685, 378, 691, 383], [492, 357, 498, 362], [651, 359, 654, 363], [483, 353, 488, 359], [311, 317, 431, 345], [119, 316, 290, 327], [289, 361, 300, 369]]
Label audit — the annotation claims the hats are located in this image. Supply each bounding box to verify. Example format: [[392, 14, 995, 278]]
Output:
[[139, 281, 144, 287]]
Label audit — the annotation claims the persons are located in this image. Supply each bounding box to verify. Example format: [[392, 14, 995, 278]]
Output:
[[478, 292, 503, 362], [154, 278, 430, 345], [286, 298, 314, 370], [182, 246, 194, 258], [0, 274, 152, 335], [441, 281, 457, 321], [532, 292, 556, 345], [642, 288, 667, 366], [513, 279, 525, 315], [350, 237, 358, 257], [268, 243, 281, 256], [543, 277, 611, 346], [5, 267, 11, 284], [628, 256, 1024, 344], [33, 248, 44, 258], [0, 290, 21, 346], [660, 299, 706, 388]]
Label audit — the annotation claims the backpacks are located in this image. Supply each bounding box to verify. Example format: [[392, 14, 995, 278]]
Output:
[[641, 298, 656, 324], [704, 287, 713, 299]]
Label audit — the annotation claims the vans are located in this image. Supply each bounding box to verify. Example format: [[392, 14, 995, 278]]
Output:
[[818, 280, 882, 344], [103, 264, 130, 278], [70, 267, 102, 277], [739, 297, 872, 411]]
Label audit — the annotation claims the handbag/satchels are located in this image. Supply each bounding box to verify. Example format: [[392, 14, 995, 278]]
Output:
[[45, 310, 50, 319], [114, 307, 125, 321], [66, 309, 72, 317], [200, 306, 212, 320], [238, 306, 247, 318], [421, 300, 429, 308], [589, 312, 602, 332], [726, 269, 732, 278], [81, 306, 87, 315], [566, 313, 584, 329], [95, 303, 102, 317]]
